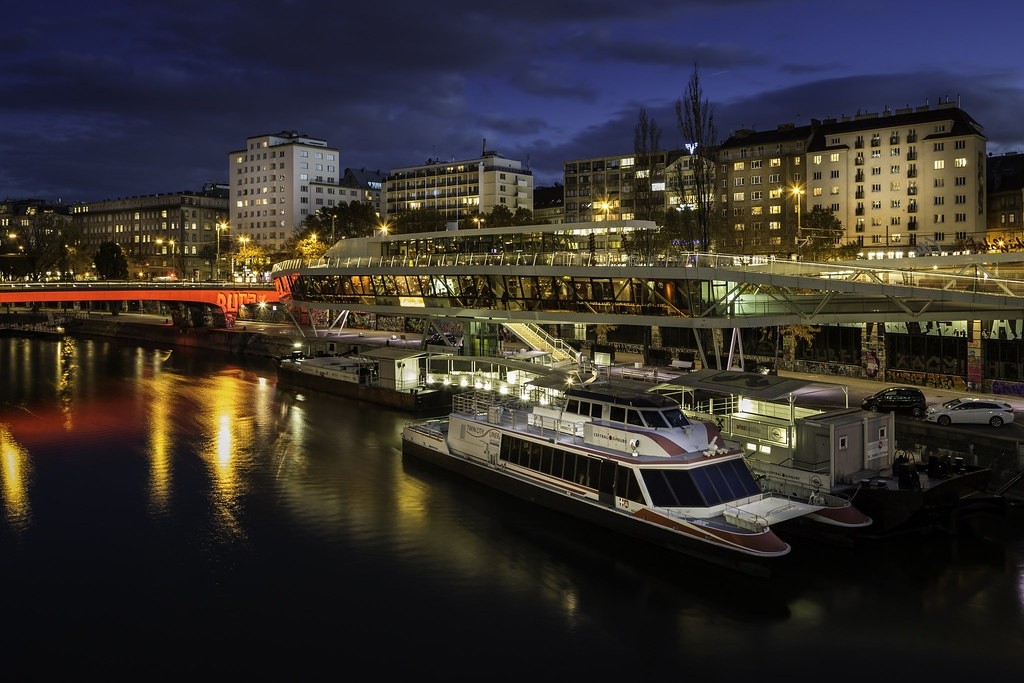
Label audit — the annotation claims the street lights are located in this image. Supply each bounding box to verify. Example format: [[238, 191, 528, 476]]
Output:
[[312, 235, 316, 257], [474, 219, 485, 229], [170, 241, 174, 278], [793, 188, 805, 235], [217, 224, 226, 280], [239, 237, 249, 282], [602, 203, 607, 222], [333, 215, 336, 246]]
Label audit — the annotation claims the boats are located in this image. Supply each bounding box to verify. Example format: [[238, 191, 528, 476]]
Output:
[[401, 381, 874, 556], [271, 346, 441, 411], [0, 320, 66, 335]]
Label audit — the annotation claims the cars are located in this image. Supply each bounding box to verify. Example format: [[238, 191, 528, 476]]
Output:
[[425, 332, 456, 347], [861, 387, 926, 417], [925, 396, 1015, 427]]
